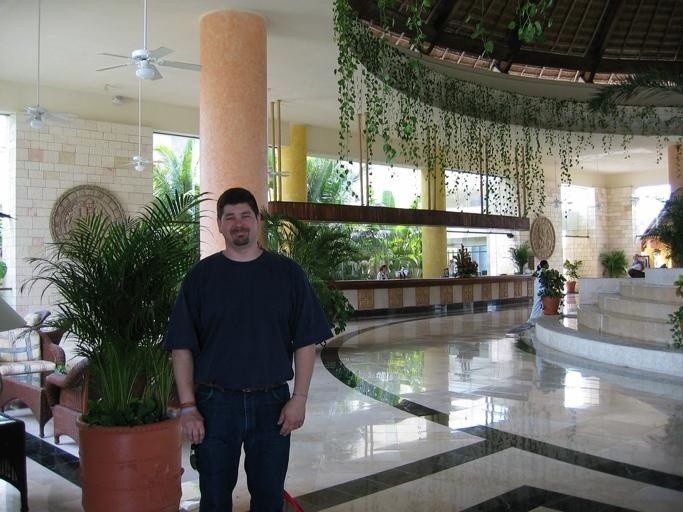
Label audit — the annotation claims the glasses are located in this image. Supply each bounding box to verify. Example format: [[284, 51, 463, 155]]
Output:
[[191, 443, 199, 470]]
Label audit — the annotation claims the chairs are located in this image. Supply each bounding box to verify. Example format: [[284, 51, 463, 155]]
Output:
[[0, 327, 148, 455]]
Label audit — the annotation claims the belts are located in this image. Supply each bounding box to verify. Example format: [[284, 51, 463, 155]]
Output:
[[196, 380, 287, 393]]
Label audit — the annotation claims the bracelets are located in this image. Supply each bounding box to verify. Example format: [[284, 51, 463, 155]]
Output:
[[292, 393, 307, 400], [178, 400, 196, 412]]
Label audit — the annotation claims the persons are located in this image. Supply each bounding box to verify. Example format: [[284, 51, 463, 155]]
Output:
[[164, 187, 335, 512], [631, 254, 644, 273], [527, 260, 549, 327], [376, 264, 388, 279]]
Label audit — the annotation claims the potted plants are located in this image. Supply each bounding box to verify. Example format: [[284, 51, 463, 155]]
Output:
[[10, 184, 217, 511], [562, 258, 582, 294], [531, 268, 566, 315]]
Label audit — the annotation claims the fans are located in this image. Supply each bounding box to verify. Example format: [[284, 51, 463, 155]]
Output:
[[17, 1, 201, 172]]
